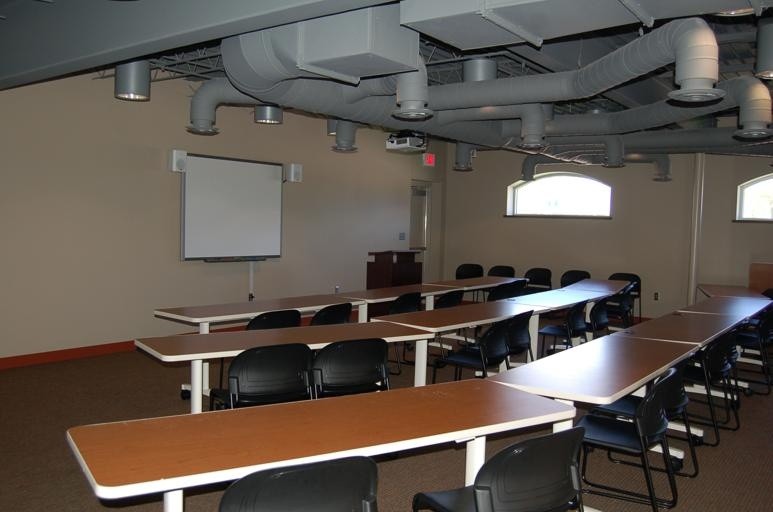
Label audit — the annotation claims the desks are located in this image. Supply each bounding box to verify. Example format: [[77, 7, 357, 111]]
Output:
[[134, 319, 436, 413], [63, 377, 577, 512], [153, 294, 366, 401]]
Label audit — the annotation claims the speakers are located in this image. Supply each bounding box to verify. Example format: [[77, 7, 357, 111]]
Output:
[[168, 150, 187, 172], [287, 164, 302, 182]]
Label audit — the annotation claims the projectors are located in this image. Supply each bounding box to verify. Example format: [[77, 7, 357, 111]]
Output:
[[385, 137, 427, 150]]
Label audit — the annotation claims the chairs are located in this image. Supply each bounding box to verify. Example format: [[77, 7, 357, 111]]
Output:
[[410, 423, 589, 511], [217, 452, 384, 511], [335, 258, 650, 376], [489, 278, 771, 510], [223, 299, 393, 400]]
